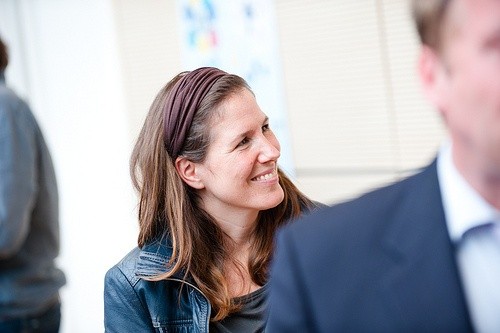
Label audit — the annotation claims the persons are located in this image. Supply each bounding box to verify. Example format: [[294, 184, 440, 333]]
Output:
[[263, 0, 500, 333], [0, 38, 66, 333], [105, 67, 330, 333]]
